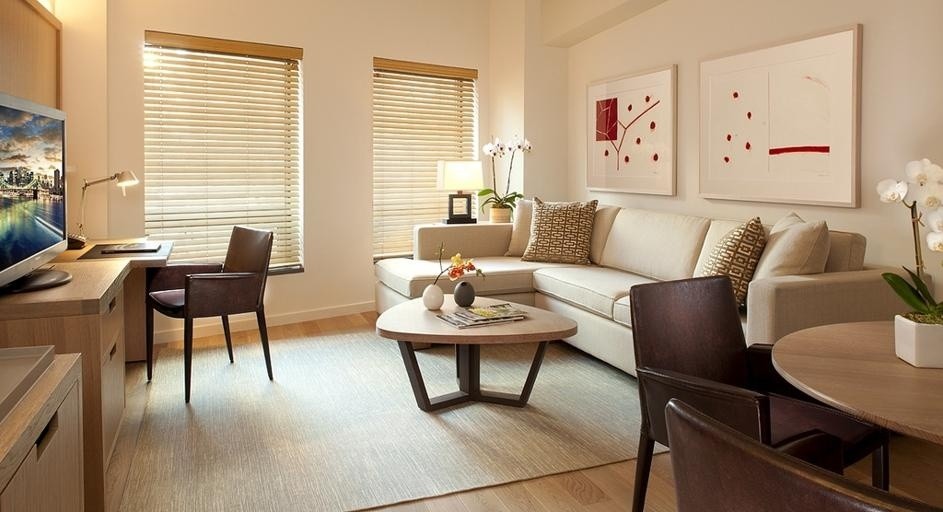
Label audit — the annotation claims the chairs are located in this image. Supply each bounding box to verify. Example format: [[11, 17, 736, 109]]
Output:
[[617, 270, 896, 510], [657, 388, 933, 511], [141, 227, 277, 407]]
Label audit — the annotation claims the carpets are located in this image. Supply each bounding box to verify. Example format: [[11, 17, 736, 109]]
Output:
[[112, 327, 670, 511]]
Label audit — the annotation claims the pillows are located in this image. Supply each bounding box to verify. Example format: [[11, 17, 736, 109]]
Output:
[[702, 214, 764, 318], [504, 195, 536, 260], [519, 196, 598, 269], [756, 205, 834, 279]]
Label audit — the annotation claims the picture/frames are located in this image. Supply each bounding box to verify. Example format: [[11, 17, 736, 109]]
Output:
[[582, 59, 680, 198], [692, 17, 862, 208]]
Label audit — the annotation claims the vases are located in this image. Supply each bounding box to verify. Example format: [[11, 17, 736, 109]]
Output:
[[889, 307, 941, 372], [485, 204, 512, 225], [420, 282, 448, 313], [451, 277, 473, 307]]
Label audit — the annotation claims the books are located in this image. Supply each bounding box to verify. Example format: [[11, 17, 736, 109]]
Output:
[[101, 243, 162, 253], [436, 301, 526, 330]]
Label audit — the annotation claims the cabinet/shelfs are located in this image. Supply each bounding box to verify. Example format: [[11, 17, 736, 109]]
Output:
[[0, 271, 129, 474], [0, 344, 89, 510]]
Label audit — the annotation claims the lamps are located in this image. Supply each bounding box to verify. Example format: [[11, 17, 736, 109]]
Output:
[[68, 167, 139, 243]]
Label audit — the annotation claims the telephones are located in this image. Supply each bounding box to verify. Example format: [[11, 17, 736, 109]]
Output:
[[67, 233, 87, 250]]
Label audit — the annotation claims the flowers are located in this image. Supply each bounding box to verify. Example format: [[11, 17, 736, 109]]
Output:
[[476, 131, 536, 210], [421, 236, 485, 285], [872, 152, 943, 322]]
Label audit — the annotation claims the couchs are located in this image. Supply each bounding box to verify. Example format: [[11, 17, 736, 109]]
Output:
[[374, 204, 919, 379]]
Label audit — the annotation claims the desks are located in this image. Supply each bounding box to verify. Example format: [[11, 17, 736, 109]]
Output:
[[45, 233, 171, 272], [767, 316, 942, 489]]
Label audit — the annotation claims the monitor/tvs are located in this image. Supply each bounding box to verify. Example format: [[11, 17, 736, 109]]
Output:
[[0, 92, 72, 295]]
